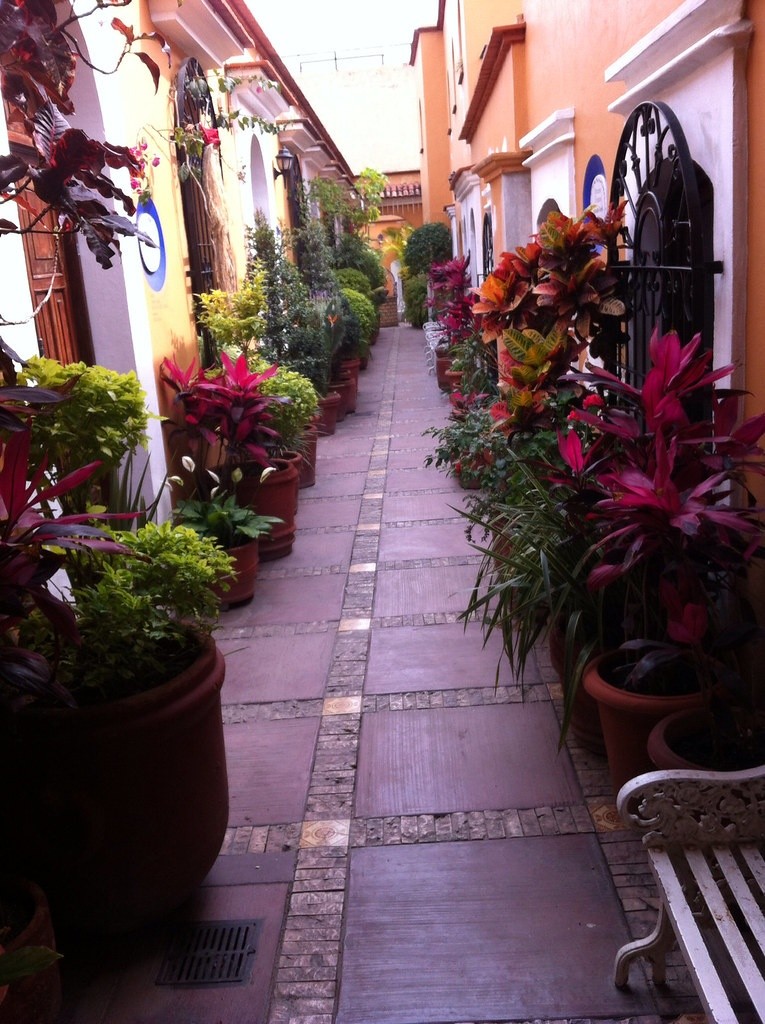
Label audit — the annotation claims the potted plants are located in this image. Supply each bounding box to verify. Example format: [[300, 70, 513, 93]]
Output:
[[0, 207, 389, 1024], [426, 256, 765, 823]]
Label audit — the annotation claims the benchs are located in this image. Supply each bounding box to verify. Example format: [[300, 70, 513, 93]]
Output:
[[422, 322, 447, 374], [615, 764, 765, 1024]]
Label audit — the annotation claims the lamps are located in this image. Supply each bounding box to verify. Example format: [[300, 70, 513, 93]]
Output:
[[273, 145, 294, 187]]
